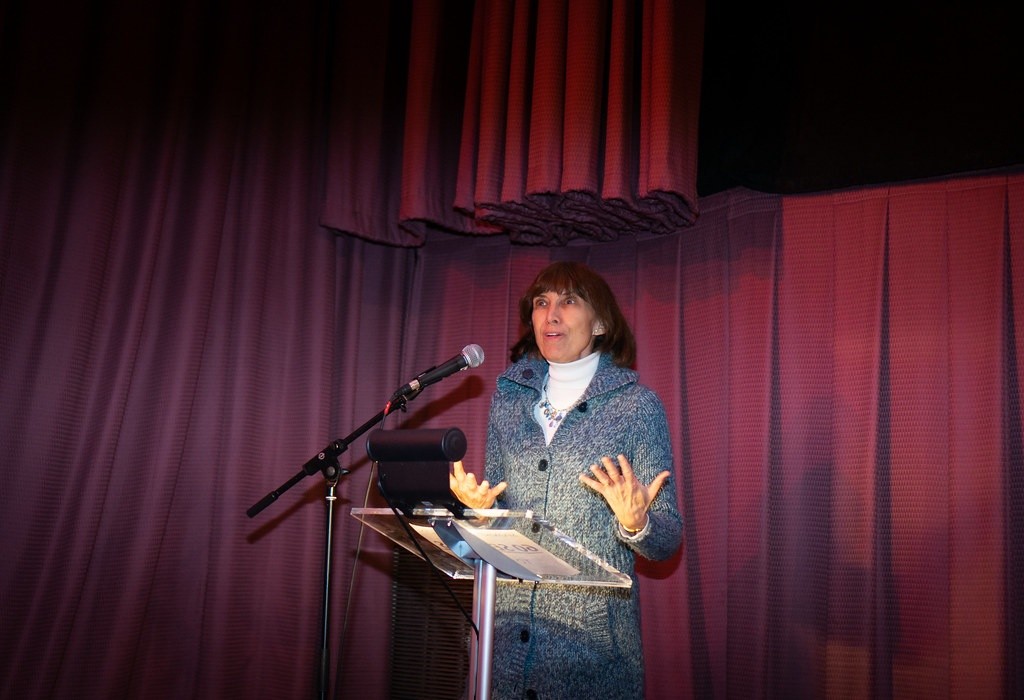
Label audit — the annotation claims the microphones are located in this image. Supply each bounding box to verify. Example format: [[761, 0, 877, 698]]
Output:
[[395, 344, 484, 398]]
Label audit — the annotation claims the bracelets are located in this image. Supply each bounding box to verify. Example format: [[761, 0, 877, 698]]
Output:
[[622, 525, 643, 533]]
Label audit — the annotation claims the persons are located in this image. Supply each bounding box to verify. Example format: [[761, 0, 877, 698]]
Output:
[[448, 260, 684, 700]]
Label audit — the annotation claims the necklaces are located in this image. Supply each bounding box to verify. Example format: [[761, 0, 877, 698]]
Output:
[[538, 374, 571, 427]]
